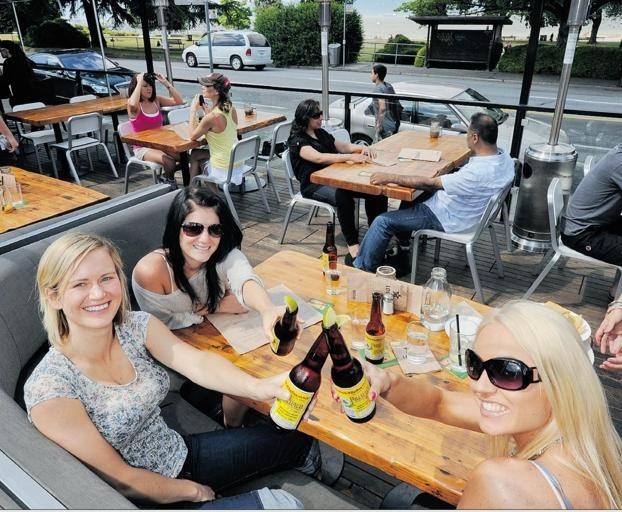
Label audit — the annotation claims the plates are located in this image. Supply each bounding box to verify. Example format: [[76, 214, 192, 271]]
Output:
[[536, 302, 596, 367], [444, 313, 484, 339]]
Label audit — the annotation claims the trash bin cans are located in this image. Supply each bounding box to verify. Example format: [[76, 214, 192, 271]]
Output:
[[510, 142, 579, 255], [328, 43, 341, 67]]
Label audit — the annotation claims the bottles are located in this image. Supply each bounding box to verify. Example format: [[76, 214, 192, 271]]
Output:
[[269, 330, 330, 430], [320, 220, 336, 275], [321, 310, 377, 425], [419, 266, 452, 331], [269, 296, 298, 357], [363, 291, 386, 364]]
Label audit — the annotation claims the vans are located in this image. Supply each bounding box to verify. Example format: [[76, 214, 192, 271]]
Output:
[[181, 29, 274, 70]]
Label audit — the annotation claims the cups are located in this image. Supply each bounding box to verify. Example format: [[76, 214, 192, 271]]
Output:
[[120, 88, 127, 99], [430, 120, 443, 139], [0, 165, 23, 215], [404, 320, 430, 364], [447, 318, 477, 370], [244, 102, 252, 116], [198, 112, 206, 123], [323, 262, 397, 353]]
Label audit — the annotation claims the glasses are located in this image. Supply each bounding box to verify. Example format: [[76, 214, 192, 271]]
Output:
[[180, 222, 227, 238], [143, 73, 156, 79], [464, 348, 542, 391], [311, 111, 324, 119]]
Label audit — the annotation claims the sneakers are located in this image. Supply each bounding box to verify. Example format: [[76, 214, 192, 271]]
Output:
[[156, 174, 167, 184], [166, 177, 179, 191], [397, 244, 410, 258]]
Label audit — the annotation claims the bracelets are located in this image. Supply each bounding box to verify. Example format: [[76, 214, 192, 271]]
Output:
[[167, 85, 171, 89]]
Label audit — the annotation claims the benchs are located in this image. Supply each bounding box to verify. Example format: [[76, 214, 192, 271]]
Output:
[[1, 181, 353, 509], [427, 58, 490, 71]]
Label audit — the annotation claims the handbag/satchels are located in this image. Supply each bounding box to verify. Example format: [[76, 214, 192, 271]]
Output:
[[385, 82, 403, 121]]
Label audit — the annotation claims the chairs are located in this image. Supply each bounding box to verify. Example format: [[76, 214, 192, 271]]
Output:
[[69, 94, 120, 171], [116, 121, 179, 195], [49, 112, 120, 188], [13, 103, 80, 174], [168, 106, 208, 170], [328, 129, 389, 213], [249, 120, 294, 204], [410, 148, 524, 306], [193, 134, 272, 230], [521, 156, 622, 307], [279, 148, 359, 244]]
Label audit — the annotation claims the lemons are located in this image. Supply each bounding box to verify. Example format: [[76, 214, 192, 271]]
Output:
[[322, 307, 336, 329], [335, 315, 350, 328], [284, 295, 298, 313]]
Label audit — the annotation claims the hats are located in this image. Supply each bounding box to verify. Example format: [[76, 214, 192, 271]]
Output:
[[197, 72, 231, 89]]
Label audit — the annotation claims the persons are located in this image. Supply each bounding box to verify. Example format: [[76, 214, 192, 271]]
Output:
[[130, 186, 306, 353], [366, 64, 404, 148], [1, 43, 39, 110], [352, 112, 517, 274], [506, 41, 512, 49], [328, 298, 621, 509], [188, 73, 246, 205], [594, 273, 621, 374], [554, 141, 622, 301], [21, 231, 323, 510], [1, 109, 20, 167], [125, 71, 184, 192], [284, 99, 390, 268], [488, 37, 503, 73]]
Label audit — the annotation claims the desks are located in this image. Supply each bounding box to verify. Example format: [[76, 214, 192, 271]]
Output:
[[1, 162, 108, 235], [120, 107, 287, 190], [309, 129, 473, 242], [165, 248, 593, 508], [4, 94, 130, 177]]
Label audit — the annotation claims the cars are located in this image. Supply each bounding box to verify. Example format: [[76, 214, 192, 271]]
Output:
[[329, 80, 572, 185], [25, 48, 136, 102]]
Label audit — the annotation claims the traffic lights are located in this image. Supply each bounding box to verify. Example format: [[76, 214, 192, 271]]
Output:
[[163, 7, 170, 22]]
